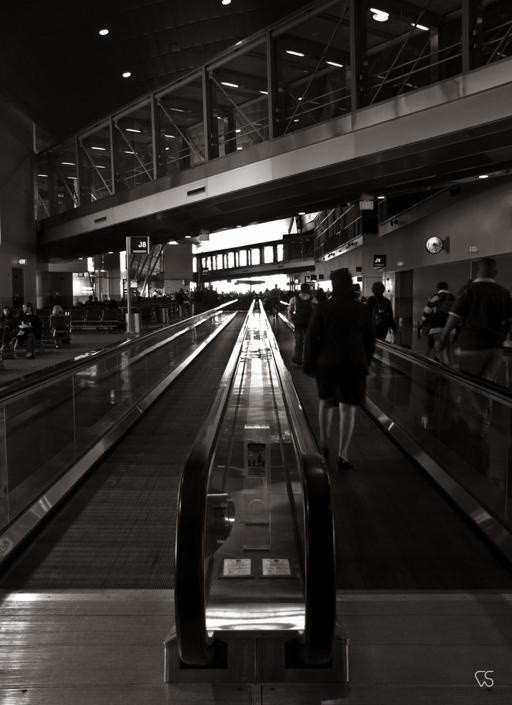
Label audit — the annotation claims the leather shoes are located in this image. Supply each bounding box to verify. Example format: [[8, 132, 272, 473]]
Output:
[[292, 356, 302, 364]]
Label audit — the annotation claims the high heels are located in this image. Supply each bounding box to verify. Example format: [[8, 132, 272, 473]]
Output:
[[337, 455, 353, 472], [318, 439, 329, 455]]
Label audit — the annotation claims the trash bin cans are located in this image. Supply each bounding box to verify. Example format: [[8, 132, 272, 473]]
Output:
[[159, 307, 169, 324], [125, 311, 142, 334]]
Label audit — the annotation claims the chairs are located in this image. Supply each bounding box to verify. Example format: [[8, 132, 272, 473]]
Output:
[[9, 307, 121, 359]]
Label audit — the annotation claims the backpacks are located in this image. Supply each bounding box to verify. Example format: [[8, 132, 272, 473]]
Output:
[[295, 295, 313, 326], [372, 295, 394, 328], [437, 292, 456, 315]]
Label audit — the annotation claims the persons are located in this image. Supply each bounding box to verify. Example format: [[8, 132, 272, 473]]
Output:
[[287, 283, 319, 365], [1, 284, 370, 362], [303, 268, 374, 470], [416, 281, 456, 366], [367, 282, 396, 369], [433, 256, 512, 382]]
